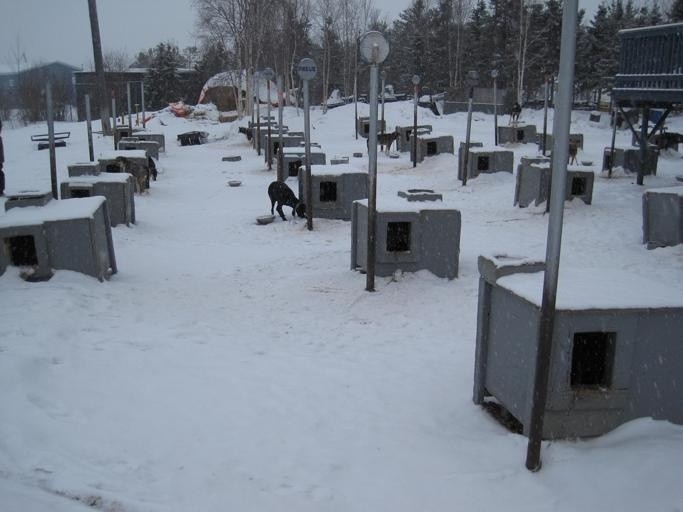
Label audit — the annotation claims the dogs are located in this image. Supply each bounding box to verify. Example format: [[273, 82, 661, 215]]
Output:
[[367, 132, 401, 154], [148, 156, 158, 181], [510, 102, 521, 122], [113, 155, 150, 195], [268, 182, 307, 221]]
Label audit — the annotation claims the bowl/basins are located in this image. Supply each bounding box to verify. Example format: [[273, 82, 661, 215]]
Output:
[[256, 214, 276, 225], [227, 180, 241, 187]]
